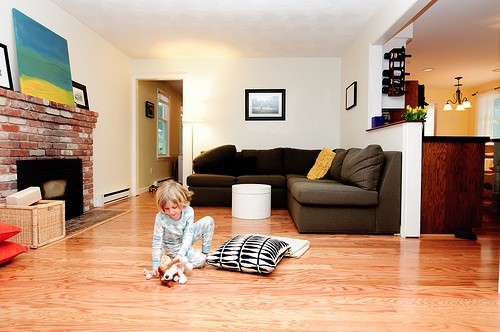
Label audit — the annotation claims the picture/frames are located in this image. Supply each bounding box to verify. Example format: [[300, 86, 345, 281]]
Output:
[[345, 81, 357, 110], [72, 80, 89, 111], [0, 43, 14, 91], [245, 88, 286, 120], [146, 101, 155, 118]]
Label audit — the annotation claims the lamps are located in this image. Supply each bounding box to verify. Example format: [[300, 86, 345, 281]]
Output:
[[442, 77, 473, 112]]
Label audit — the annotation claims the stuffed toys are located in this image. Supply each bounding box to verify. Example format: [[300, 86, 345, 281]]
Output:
[[158, 255, 188, 287]]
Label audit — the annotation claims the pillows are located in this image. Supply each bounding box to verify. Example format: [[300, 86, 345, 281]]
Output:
[[308, 149, 336, 180], [341, 144, 385, 190], [193, 145, 237, 173], [206, 234, 290, 275], [0, 221, 31, 266]]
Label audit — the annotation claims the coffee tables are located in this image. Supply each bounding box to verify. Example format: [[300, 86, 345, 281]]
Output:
[[232, 183, 271, 220]]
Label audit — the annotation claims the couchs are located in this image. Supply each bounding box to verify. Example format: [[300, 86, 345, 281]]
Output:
[[186, 148, 402, 237]]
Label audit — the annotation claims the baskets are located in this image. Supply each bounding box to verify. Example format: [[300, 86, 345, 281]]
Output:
[[0, 200, 66, 248]]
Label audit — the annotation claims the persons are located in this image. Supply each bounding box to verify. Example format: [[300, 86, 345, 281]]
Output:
[[150, 180, 215, 278]]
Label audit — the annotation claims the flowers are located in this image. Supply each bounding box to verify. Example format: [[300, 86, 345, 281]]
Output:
[[400, 105, 428, 121]]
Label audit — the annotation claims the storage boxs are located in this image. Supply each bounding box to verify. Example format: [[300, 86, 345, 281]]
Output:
[[5, 187, 42, 206]]
